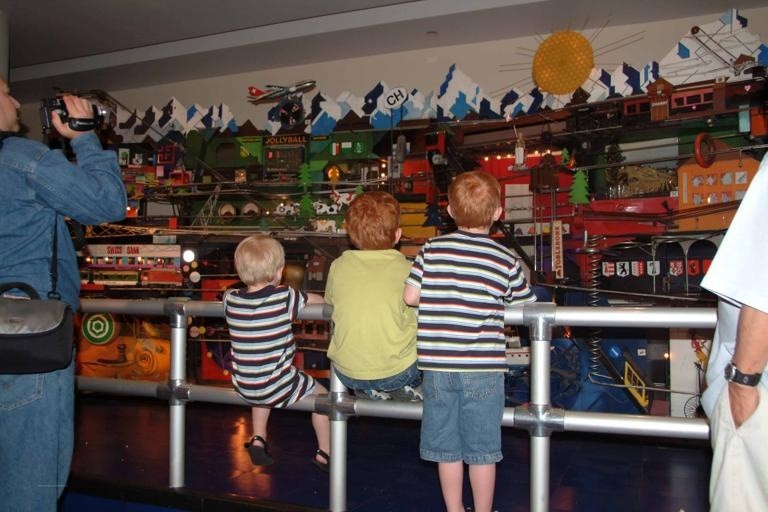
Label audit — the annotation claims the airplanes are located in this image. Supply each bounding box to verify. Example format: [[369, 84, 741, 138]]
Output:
[[246, 79, 316, 106]]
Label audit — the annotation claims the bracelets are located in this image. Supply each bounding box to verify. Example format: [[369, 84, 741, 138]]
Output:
[[722, 361, 763, 387]]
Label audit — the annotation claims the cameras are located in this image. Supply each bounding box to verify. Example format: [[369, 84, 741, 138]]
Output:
[[38, 96, 109, 135]]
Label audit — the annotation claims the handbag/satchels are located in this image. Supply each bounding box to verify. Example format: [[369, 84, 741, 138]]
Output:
[[0, 296, 75, 374]]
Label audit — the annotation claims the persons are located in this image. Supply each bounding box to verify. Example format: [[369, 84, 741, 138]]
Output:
[[322, 189, 424, 407], [697, 146, 768, 511], [0, 73, 132, 512], [401, 168, 538, 511], [220, 230, 331, 469]]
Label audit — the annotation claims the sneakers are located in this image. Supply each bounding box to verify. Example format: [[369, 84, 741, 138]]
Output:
[[354, 386, 424, 403]]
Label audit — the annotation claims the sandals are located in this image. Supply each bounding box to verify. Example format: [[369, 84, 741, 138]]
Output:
[[247, 436, 274, 466], [314, 449, 329, 471]]
